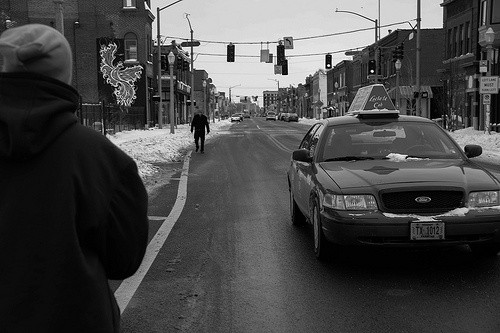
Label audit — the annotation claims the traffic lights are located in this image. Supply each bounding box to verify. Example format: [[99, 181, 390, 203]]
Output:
[[397, 46, 404, 59], [227, 45, 234, 62], [392, 50, 397, 62], [369, 60, 376, 74], [277, 45, 286, 66], [421, 91, 428, 99], [281, 60, 288, 75], [325, 55, 332, 69]]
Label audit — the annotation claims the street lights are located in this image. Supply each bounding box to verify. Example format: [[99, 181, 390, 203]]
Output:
[[335, 9, 379, 84], [484, 27, 495, 135], [167, 51, 175, 135], [395, 58, 402, 110]]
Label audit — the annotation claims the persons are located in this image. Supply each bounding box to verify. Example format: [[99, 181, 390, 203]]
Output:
[[190, 109, 210, 153], [0, 23, 149, 333]]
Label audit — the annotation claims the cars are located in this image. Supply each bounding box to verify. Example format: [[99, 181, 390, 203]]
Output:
[[286, 83, 500, 264], [278, 112, 287, 121], [235, 113, 243, 121], [284, 113, 298, 122], [243, 113, 251, 118], [231, 114, 241, 122]]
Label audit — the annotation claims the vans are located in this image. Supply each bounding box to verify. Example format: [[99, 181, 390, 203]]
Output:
[[266, 111, 276, 121]]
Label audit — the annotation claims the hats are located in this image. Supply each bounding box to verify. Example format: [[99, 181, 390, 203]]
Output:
[[0, 24, 73, 85]]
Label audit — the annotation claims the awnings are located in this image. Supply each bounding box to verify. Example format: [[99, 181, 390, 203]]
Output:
[[399, 86, 433, 99], [387, 88, 396, 99]]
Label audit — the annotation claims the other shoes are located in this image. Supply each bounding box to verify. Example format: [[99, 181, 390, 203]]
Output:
[[201, 150, 205, 154], [195, 146, 199, 153]]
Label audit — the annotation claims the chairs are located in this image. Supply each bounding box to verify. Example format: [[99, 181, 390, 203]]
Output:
[[325, 133, 360, 158]]
